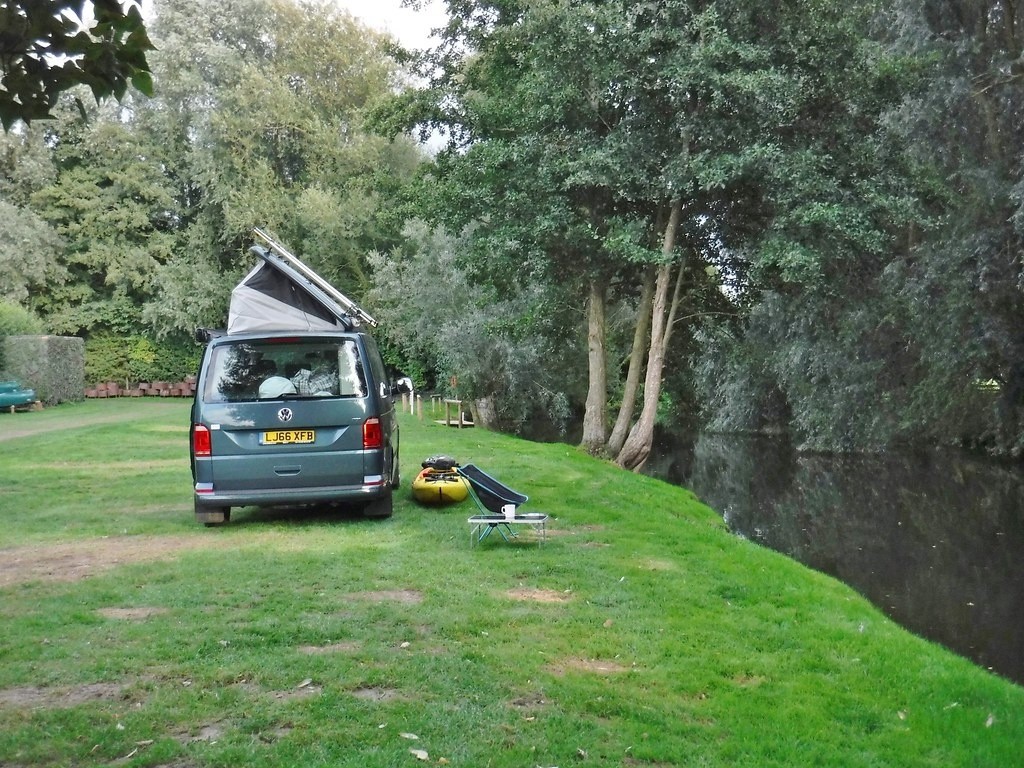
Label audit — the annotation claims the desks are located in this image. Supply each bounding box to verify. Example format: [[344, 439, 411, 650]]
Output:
[[468, 515, 549, 549]]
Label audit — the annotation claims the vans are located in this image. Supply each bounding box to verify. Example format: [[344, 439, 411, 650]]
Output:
[[188, 331, 401, 527]]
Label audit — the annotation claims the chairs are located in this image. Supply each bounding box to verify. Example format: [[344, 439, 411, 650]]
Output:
[[256, 360, 277, 383], [457, 462, 528, 543]]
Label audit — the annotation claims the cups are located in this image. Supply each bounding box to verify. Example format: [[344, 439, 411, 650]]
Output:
[[502, 504, 515, 519]]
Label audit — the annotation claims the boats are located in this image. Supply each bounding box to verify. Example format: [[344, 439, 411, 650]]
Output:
[[411, 465, 470, 506]]
